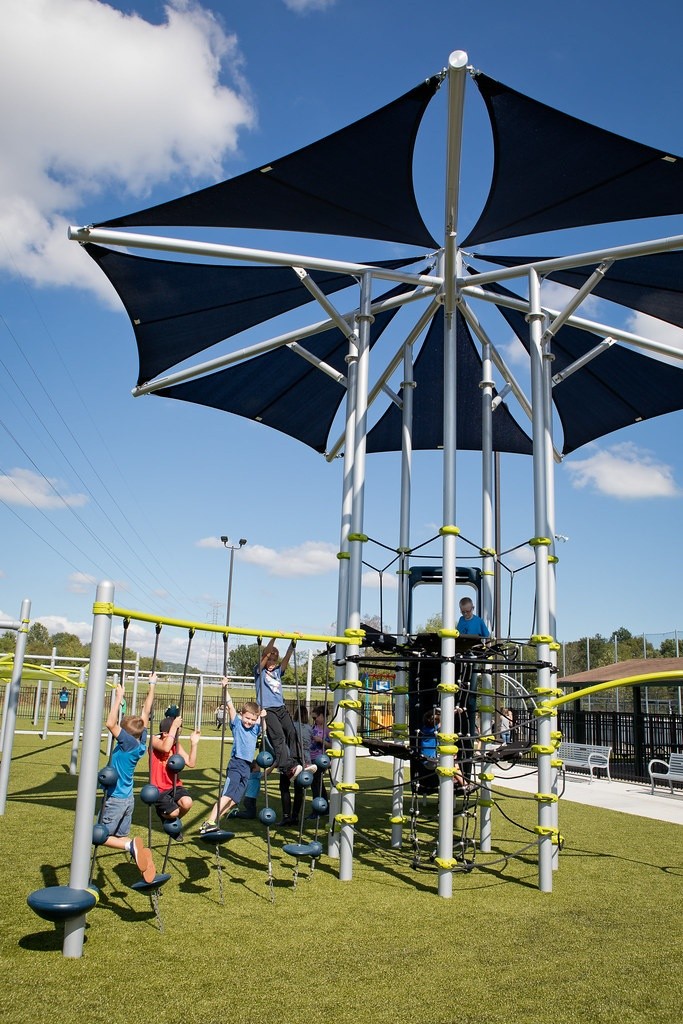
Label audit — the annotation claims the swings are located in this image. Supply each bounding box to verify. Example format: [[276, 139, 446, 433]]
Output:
[[215, 677, 229, 725]]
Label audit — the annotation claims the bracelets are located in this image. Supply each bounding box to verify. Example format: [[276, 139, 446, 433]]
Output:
[[167, 733, 176, 738], [148, 681, 157, 685]]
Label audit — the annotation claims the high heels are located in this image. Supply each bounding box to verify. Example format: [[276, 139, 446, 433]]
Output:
[[276, 817, 291, 826], [291, 817, 299, 826]]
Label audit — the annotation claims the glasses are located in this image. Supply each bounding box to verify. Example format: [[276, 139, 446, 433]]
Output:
[[312, 714, 321, 720], [460, 607, 473, 614]]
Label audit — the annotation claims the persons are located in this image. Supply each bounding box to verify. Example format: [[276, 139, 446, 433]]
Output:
[[475, 721, 480, 745], [456, 597, 492, 639], [96, 674, 158, 884], [55, 684, 128, 721], [500, 708, 511, 747], [418, 708, 473, 790], [199, 630, 332, 833], [148, 717, 201, 840]]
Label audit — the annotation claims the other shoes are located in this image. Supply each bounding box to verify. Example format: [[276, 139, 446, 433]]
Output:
[[227, 808, 240, 819], [464, 783, 479, 794], [170, 832, 184, 843], [320, 812, 329, 817], [286, 764, 302, 783], [140, 849, 157, 883], [130, 837, 147, 872], [216, 727, 220, 731], [305, 813, 320, 820], [59, 713, 66, 720], [304, 763, 318, 774], [199, 819, 218, 833]]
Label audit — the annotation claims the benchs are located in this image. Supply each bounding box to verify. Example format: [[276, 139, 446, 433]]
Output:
[[558, 742, 612, 785], [648, 753, 683, 795]]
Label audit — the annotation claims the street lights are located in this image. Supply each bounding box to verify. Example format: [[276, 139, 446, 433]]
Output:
[[221, 536, 247, 709]]
